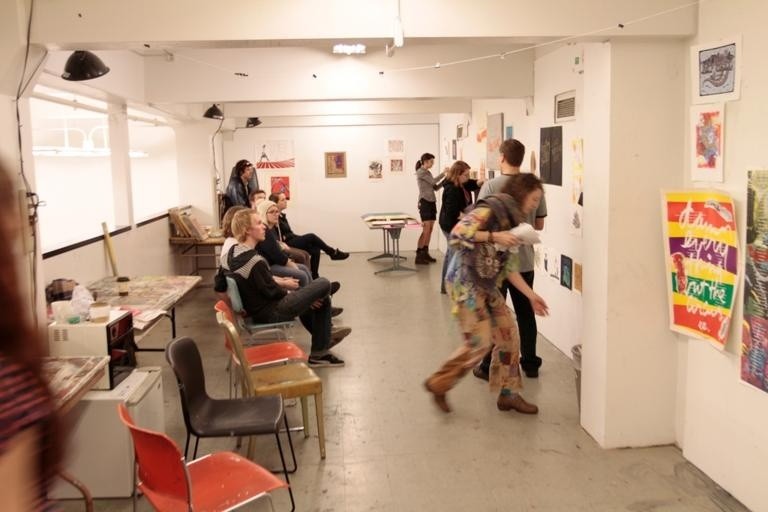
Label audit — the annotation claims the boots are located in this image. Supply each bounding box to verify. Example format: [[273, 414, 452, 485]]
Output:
[[415, 248, 429, 264], [422, 245, 435, 262]]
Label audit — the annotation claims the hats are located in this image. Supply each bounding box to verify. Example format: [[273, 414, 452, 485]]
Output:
[[256, 198, 277, 226]]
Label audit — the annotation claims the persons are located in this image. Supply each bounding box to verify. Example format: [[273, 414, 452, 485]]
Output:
[[415, 153, 448, 264], [218, 158, 352, 368], [423, 171, 550, 413], [439, 161, 487, 294], [1, 166, 54, 512], [472, 136, 547, 381]]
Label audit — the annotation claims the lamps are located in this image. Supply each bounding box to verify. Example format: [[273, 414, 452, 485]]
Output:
[[202, 104, 224, 121], [61, 50, 110, 82], [245, 118, 263, 130]]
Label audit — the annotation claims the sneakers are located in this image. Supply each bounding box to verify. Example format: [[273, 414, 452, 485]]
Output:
[[332, 326, 351, 337], [330, 307, 343, 316], [331, 282, 340, 295], [331, 248, 349, 260], [308, 354, 344, 368]]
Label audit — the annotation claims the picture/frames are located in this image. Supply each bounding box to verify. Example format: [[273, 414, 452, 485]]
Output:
[[324, 152, 347, 179], [690, 35, 741, 105], [689, 103, 724, 184]]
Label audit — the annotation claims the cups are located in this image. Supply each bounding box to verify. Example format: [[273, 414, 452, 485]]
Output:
[[116, 277, 130, 296], [89, 302, 111, 323], [50, 301, 71, 324]]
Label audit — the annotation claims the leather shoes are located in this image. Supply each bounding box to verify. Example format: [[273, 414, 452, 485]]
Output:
[[425, 379, 450, 411], [473, 366, 489, 379], [497, 394, 538, 414]]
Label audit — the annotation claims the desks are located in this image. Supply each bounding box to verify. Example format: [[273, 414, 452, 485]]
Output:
[[170, 232, 227, 290], [360, 212, 423, 276], [40, 355, 112, 512], [85, 273, 204, 352]]
